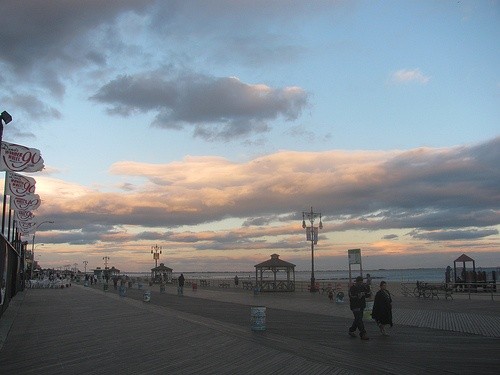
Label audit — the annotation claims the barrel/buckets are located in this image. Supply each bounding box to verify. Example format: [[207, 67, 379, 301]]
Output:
[[76, 278, 142, 297], [251, 306, 266, 331], [160, 285, 165, 293], [144, 290, 150, 302]]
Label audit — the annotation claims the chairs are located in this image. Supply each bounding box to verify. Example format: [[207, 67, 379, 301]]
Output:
[[30, 280, 71, 288]]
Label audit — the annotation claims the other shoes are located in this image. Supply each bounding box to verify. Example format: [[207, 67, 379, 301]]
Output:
[[349, 330, 358, 337], [360, 334, 369, 339]]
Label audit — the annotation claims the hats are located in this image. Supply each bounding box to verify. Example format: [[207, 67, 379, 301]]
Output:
[[356, 276, 364, 281]]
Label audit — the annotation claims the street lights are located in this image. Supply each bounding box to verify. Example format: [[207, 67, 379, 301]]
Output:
[[301, 206, 323, 292], [102, 256, 110, 279], [150, 244, 162, 280], [83, 260, 89, 277], [31, 221, 56, 280], [0, 110, 13, 155]]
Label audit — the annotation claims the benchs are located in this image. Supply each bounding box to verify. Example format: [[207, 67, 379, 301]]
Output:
[[243, 281, 254, 290], [219, 283, 230, 288], [199, 279, 208, 288]]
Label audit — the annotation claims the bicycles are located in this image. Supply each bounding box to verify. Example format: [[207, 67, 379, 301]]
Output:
[[326, 286, 345, 303], [413, 281, 438, 298]]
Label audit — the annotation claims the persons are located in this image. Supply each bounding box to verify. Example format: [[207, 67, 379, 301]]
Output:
[[159, 273, 168, 286], [39, 272, 71, 281], [372, 281, 393, 335], [178, 274, 185, 288], [105, 273, 129, 287], [84, 274, 98, 287], [348, 276, 371, 340]]
